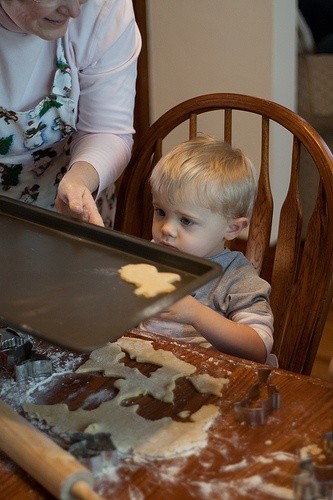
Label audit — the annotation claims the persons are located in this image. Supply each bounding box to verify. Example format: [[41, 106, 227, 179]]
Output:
[[0, 0, 141, 231], [133, 135, 274, 364]]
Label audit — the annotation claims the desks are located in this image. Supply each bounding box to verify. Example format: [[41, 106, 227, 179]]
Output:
[[0, 328, 333, 500]]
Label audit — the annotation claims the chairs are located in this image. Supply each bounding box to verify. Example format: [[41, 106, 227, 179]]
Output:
[[113, 94, 333, 377]]
